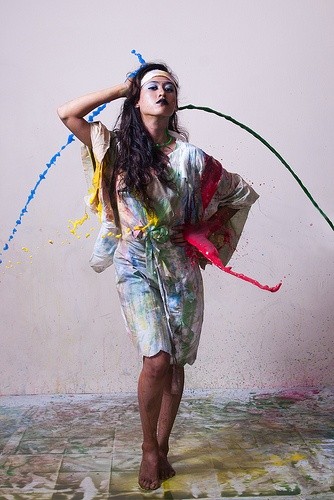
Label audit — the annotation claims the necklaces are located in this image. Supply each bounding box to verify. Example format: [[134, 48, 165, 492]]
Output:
[[154, 136, 172, 147]]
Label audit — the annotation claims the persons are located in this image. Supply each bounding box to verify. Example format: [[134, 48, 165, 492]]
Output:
[[56, 62, 260, 491]]
